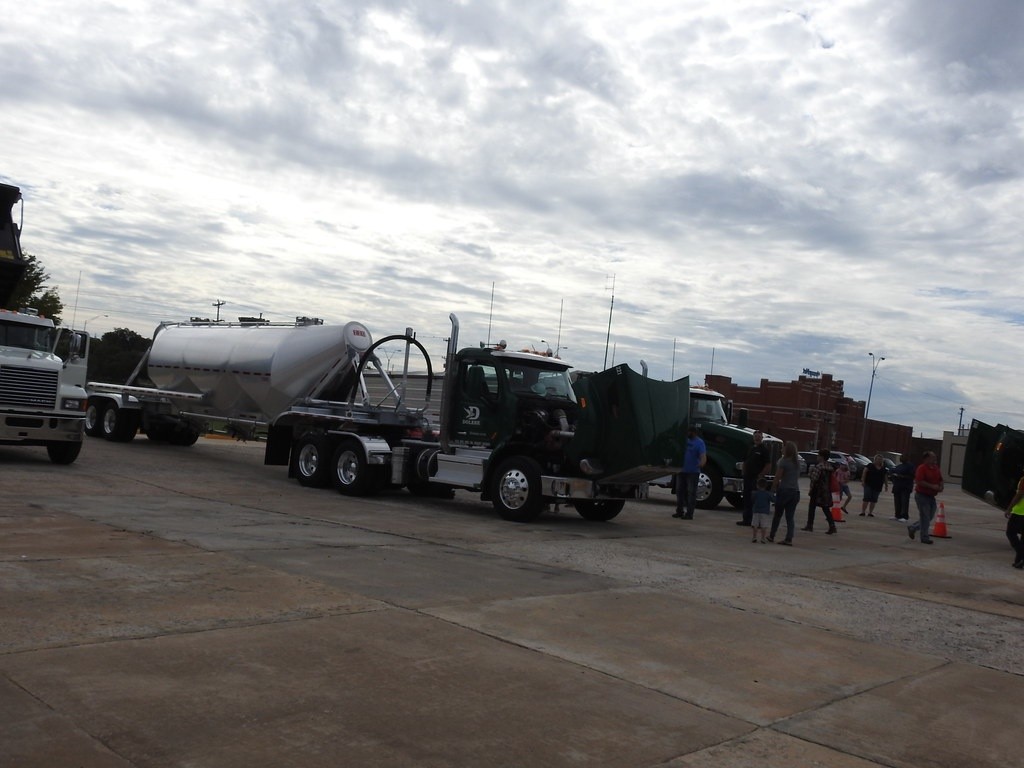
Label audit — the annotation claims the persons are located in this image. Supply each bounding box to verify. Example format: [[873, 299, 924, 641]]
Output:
[[859, 450, 944, 544], [736, 431, 852, 546], [673, 428, 706, 519], [1005, 476, 1024, 568]]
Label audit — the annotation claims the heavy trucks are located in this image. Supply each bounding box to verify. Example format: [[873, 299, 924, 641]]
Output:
[[84, 334, 691, 522], [364, 369, 785, 512]]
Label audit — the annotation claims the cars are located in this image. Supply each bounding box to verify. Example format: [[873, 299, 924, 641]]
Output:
[[796, 450, 898, 484]]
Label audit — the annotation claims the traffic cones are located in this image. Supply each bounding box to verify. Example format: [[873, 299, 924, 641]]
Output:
[[926, 502, 953, 539], [826, 491, 848, 523]]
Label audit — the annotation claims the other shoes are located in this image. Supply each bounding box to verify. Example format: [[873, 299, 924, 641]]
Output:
[[907, 525, 915, 539], [735, 520, 751, 526], [681, 512, 693, 520], [752, 536, 757, 542], [921, 539, 934, 544], [841, 506, 848, 515], [868, 513, 874, 517], [766, 536, 775, 542], [1013, 556, 1024, 568], [761, 538, 768, 543], [859, 512, 866, 516], [898, 518, 909, 522], [825, 525, 837, 534], [777, 539, 793, 546], [672, 511, 685, 517], [801, 521, 813, 532], [889, 516, 897, 520]]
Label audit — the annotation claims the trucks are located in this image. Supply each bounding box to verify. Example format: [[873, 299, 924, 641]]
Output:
[[1, 307, 91, 467]]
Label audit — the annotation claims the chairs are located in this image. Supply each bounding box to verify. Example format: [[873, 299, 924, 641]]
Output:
[[468, 367, 490, 394]]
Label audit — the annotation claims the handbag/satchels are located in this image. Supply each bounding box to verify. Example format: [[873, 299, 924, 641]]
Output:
[[831, 469, 840, 493]]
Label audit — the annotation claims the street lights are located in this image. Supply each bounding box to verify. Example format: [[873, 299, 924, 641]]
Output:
[[859, 352, 887, 455]]
